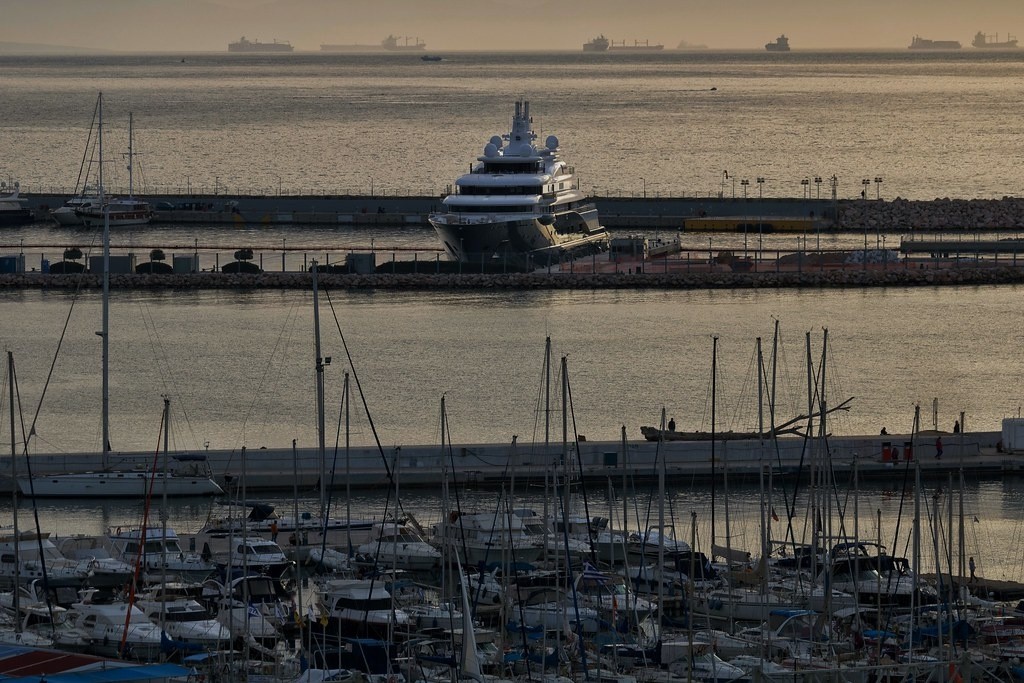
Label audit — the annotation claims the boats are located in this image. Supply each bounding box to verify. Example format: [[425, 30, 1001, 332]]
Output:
[[0, 182, 35, 225], [583, 34, 609, 51], [609, 40, 663, 50], [229, 37, 294, 52], [428, 101, 610, 269], [971, 31, 1018, 48], [908, 35, 961, 49], [320, 34, 426, 50], [765, 34, 790, 51]]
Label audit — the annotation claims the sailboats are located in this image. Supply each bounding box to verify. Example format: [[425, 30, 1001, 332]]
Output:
[[0, 259, 1023, 683], [0, 194, 226, 496], [51, 92, 112, 226], [73, 111, 154, 226]]
